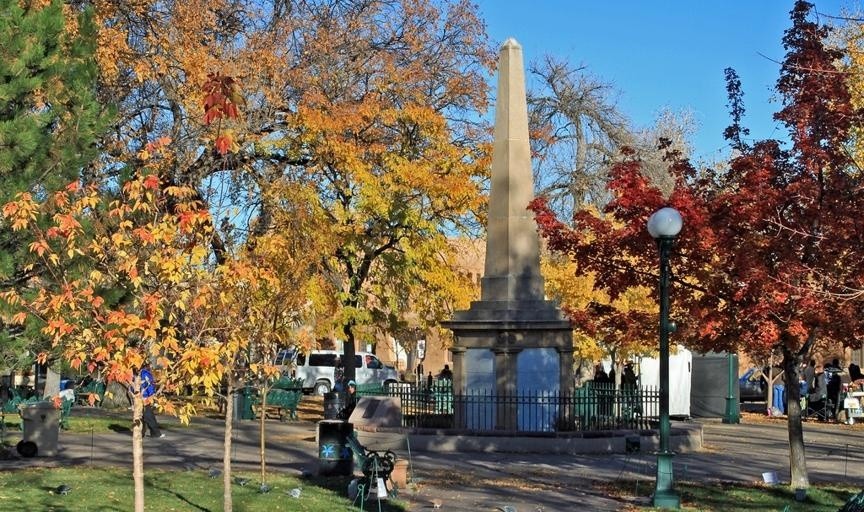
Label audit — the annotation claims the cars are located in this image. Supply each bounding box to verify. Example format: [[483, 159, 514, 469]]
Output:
[[739, 366, 768, 403]]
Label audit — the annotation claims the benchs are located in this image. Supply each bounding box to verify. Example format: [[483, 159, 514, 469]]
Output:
[[247, 387, 304, 422], [72, 380, 105, 408], [0, 394, 73, 432]]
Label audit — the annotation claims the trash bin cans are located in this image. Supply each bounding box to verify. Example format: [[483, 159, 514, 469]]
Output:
[[17, 404, 58, 456], [232, 387, 244, 422], [324, 391, 347, 419]]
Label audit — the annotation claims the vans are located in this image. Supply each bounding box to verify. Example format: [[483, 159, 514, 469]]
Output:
[[270, 346, 402, 397]]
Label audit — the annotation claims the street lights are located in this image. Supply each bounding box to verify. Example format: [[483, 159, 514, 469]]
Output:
[[647, 207, 683, 512]]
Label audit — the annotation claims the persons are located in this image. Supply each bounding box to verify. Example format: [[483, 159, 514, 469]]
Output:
[[440, 365, 452, 379], [799, 365, 828, 412], [336, 380, 356, 419], [428, 371, 432, 388], [130, 363, 165, 438], [333, 357, 346, 392], [772, 368, 785, 413]]
